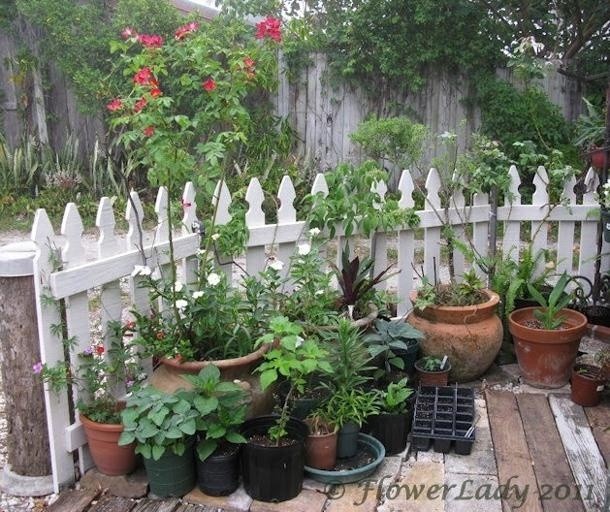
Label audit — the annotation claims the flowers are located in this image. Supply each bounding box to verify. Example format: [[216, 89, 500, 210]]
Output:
[[32, 278, 149, 420], [96, 12, 293, 360]]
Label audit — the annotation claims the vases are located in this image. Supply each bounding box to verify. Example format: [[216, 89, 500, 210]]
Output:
[[80, 399, 137, 476]]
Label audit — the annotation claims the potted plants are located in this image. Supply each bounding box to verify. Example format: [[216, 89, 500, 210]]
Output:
[[120, 220, 609, 502]]
